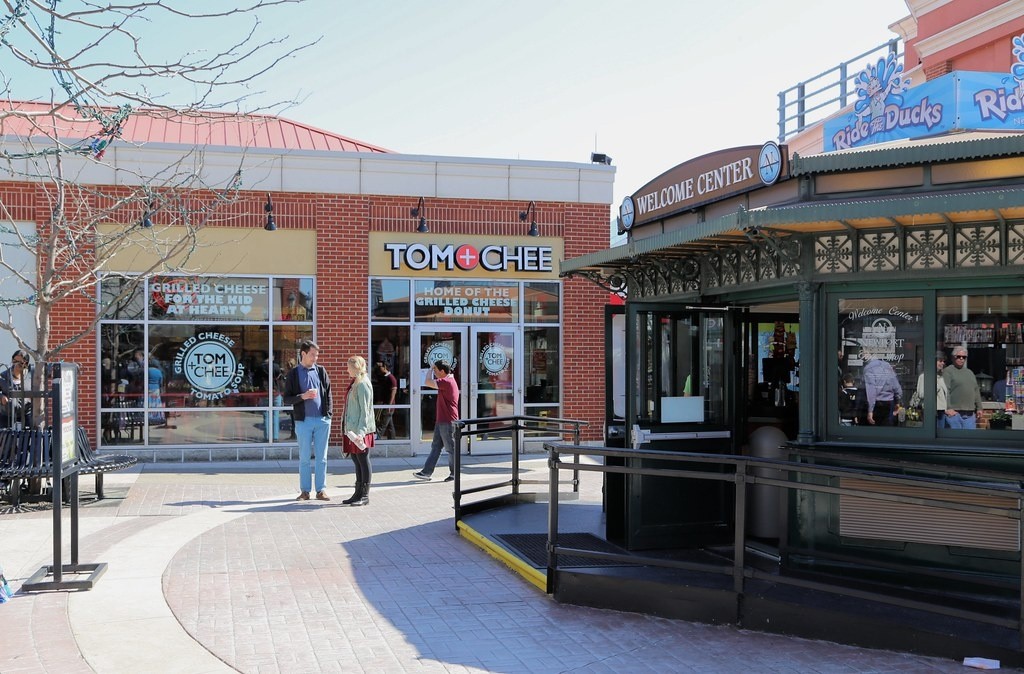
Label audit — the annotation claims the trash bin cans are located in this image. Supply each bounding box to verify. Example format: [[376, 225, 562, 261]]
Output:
[[744, 426, 790, 544]]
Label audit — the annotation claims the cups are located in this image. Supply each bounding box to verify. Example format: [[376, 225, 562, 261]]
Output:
[[309, 389, 317, 398]]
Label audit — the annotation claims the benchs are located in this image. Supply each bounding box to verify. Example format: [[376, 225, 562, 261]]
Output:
[[0, 427, 138, 507], [99, 401, 168, 444]]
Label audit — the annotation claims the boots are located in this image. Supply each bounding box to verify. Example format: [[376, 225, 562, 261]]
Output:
[[351, 482, 370, 506], [342, 482, 363, 504]]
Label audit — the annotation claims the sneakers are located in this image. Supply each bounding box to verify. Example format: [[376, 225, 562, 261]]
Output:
[[316, 490, 329, 501], [297, 492, 310, 501]]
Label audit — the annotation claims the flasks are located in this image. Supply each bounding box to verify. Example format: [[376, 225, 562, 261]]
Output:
[[347, 430, 367, 450]]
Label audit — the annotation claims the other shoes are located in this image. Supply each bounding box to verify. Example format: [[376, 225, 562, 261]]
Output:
[[444, 476, 454, 482], [413, 472, 432, 480]]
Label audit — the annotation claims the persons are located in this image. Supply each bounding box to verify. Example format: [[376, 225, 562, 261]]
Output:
[[917, 352, 948, 429], [683, 374, 690, 396], [284, 357, 298, 441], [126, 351, 166, 425], [864, 359, 902, 427], [413, 360, 460, 482], [837, 349, 869, 426], [376, 361, 398, 440], [284, 340, 333, 501], [101, 358, 111, 393], [993, 370, 1007, 402], [0, 349, 30, 490], [252, 351, 284, 443], [943, 345, 983, 429], [340, 356, 376, 506]]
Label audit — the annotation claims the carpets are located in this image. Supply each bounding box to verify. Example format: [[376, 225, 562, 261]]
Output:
[[491, 532, 645, 568]]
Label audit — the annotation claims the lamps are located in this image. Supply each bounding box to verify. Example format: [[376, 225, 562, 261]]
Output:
[[264, 193, 277, 230], [140, 199, 154, 227], [519, 201, 539, 237], [411, 197, 428, 232]]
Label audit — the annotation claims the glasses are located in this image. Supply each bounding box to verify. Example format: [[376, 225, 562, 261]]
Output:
[[956, 355, 967, 359]]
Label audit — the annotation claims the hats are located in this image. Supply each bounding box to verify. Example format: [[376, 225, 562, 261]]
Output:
[[937, 351, 947, 360]]
